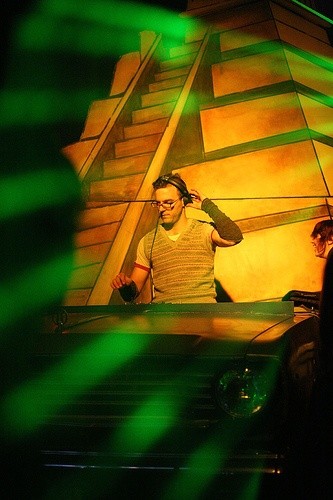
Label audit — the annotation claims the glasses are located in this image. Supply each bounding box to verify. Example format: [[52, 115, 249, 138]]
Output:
[[151, 195, 183, 208]]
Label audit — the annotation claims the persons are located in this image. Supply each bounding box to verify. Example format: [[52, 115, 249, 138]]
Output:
[[109, 172, 244, 303], [311, 220, 333, 427]]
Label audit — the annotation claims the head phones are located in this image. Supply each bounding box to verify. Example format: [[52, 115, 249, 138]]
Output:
[[155, 176, 193, 206]]
